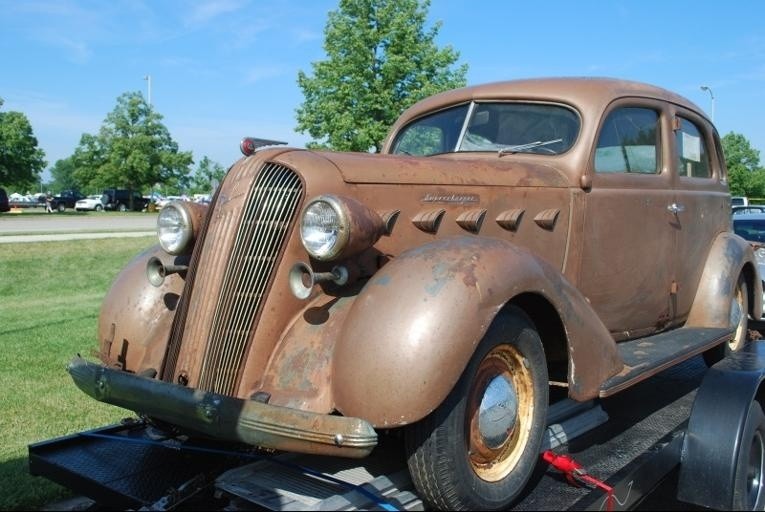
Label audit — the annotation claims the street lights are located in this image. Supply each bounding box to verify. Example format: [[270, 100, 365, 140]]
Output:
[[143, 75, 153, 203]]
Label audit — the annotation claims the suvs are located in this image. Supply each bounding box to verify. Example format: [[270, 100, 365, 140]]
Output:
[[101, 189, 156, 211]]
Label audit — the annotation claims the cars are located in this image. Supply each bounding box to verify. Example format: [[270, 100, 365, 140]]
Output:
[[66, 79, 763, 511], [75, 195, 112, 211], [143, 192, 212, 210]]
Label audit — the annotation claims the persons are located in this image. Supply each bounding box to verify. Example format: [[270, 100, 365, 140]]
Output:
[[46, 191, 53, 213]]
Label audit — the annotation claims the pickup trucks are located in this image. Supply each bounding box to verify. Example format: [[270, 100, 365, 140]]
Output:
[[45, 190, 88, 211]]
